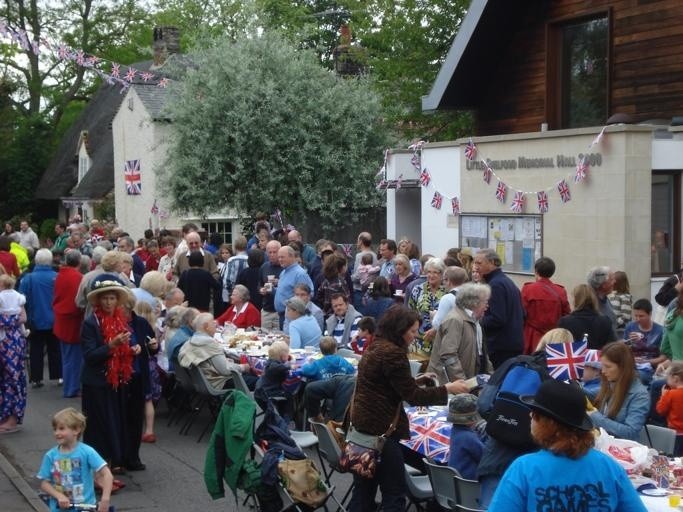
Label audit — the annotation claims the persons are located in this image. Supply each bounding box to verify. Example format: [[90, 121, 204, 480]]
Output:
[[37, 407, 114, 511], [274, 219, 683, 512], [1, 218, 301, 494]]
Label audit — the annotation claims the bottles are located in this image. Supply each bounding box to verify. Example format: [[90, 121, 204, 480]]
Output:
[[582, 333, 588, 343]]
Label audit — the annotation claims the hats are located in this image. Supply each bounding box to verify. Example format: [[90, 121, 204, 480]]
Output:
[[446, 393, 483, 425], [283, 297, 305, 314], [85, 273, 126, 305], [519, 379, 593, 431]]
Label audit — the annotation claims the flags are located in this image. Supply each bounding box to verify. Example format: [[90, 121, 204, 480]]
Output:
[[124, 161, 141, 195], [0, 22, 170, 89], [375, 132, 604, 215]]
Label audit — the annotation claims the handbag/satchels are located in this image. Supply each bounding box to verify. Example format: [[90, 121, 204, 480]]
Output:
[[339, 425, 385, 480], [484, 355, 554, 449]]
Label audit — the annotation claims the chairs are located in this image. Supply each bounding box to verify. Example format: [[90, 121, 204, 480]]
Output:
[[155, 361, 682, 511]]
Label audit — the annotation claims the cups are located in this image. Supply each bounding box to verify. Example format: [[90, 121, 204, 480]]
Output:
[[267, 275, 275, 284], [369, 282, 374, 287], [632, 332, 645, 341], [396, 289, 402, 294], [304, 345, 315, 359], [669, 495, 681, 507]]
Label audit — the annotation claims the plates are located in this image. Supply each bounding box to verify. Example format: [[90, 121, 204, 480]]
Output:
[[392, 293, 404, 296], [436, 416, 447, 422], [367, 287, 374, 289], [249, 351, 268, 358], [642, 487, 669, 497]]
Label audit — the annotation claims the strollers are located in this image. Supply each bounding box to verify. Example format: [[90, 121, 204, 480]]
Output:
[[225, 384, 347, 512]]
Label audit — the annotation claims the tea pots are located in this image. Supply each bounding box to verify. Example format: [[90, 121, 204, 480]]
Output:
[[218, 319, 237, 341]]
